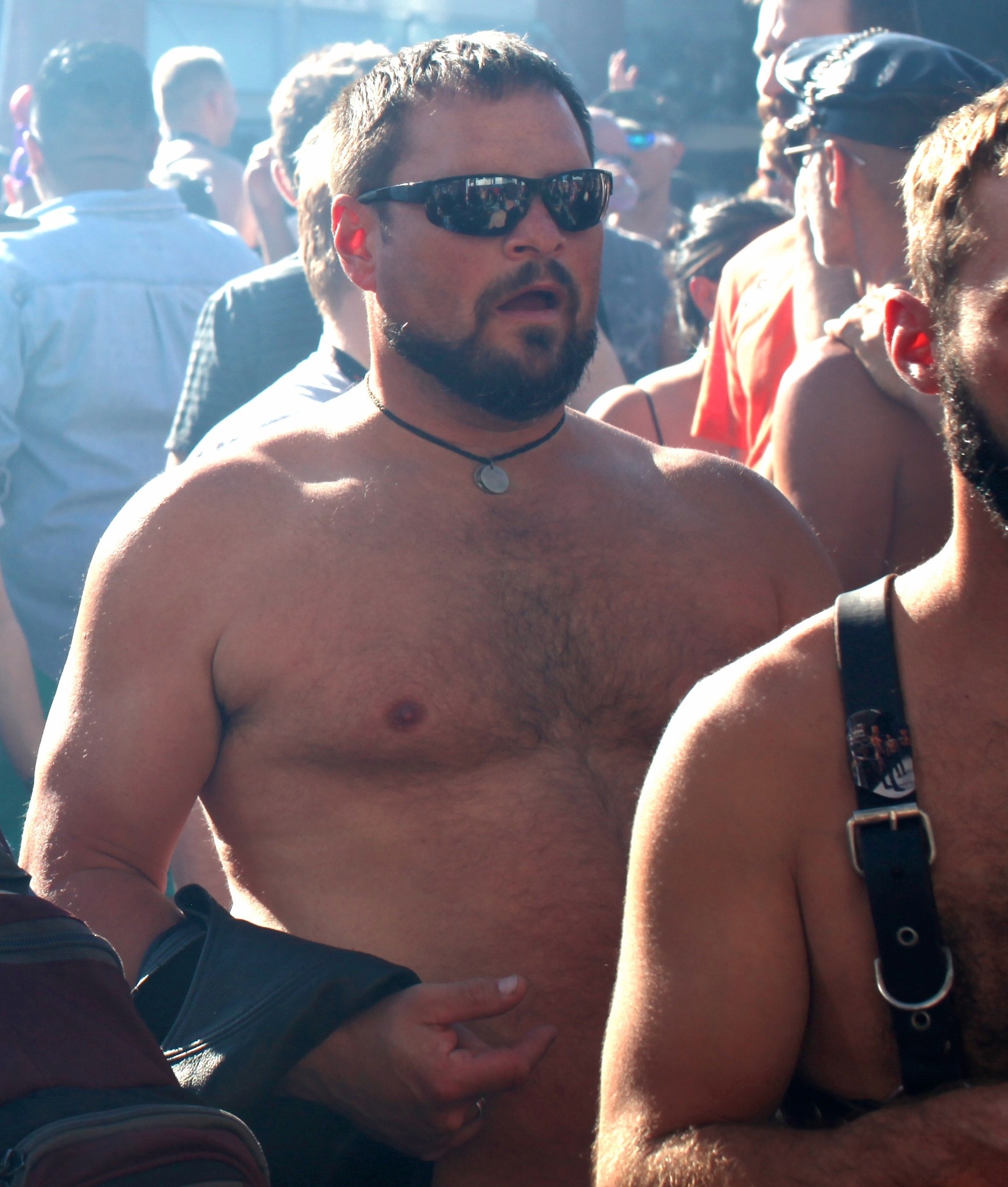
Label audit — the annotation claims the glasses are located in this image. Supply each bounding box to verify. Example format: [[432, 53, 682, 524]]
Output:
[[784, 144, 866, 171], [624, 127, 677, 152], [357, 169, 618, 235]]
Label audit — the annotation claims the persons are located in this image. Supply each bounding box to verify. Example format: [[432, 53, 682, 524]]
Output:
[[0, 1, 1007, 1187]]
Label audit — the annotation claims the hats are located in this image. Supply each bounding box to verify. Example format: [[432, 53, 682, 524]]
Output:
[[776, 31, 859, 100], [795, 26, 1002, 146]]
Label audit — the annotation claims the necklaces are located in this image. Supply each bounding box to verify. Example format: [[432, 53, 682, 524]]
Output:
[[364, 371, 566, 493]]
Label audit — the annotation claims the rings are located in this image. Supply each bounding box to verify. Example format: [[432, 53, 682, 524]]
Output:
[[474, 1094, 485, 1121]]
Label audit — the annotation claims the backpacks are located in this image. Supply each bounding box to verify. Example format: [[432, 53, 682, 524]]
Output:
[[0, 840, 270, 1187]]
